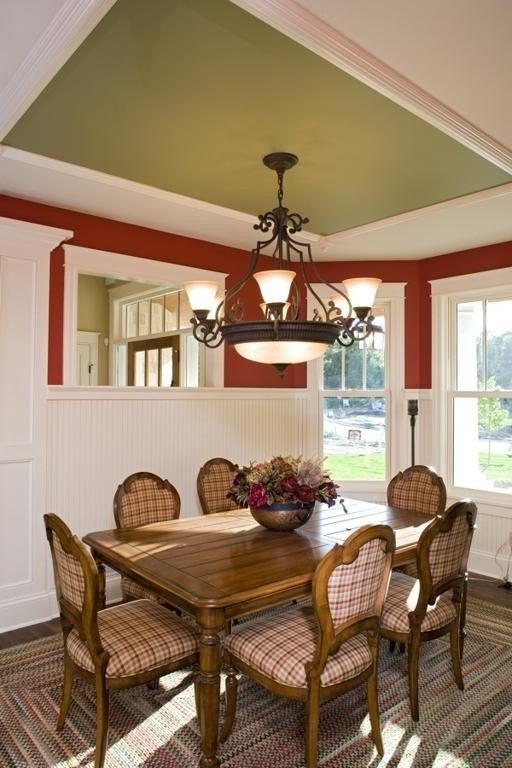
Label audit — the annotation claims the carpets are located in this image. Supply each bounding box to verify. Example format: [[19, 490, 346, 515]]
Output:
[[1, 590, 512, 768]]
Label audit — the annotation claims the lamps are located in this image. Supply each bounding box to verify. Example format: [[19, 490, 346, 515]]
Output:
[[181, 151, 382, 380]]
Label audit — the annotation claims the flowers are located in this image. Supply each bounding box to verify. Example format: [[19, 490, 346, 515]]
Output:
[[225, 452, 348, 516]]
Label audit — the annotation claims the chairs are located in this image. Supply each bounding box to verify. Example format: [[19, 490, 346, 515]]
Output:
[[196, 458, 248, 515], [388, 465, 447, 578], [379, 499, 477, 722], [112, 472, 182, 616], [43, 512, 201, 768], [219, 524, 396, 768]]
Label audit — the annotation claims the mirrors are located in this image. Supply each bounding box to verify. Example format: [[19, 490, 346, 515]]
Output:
[[62, 242, 229, 389]]
